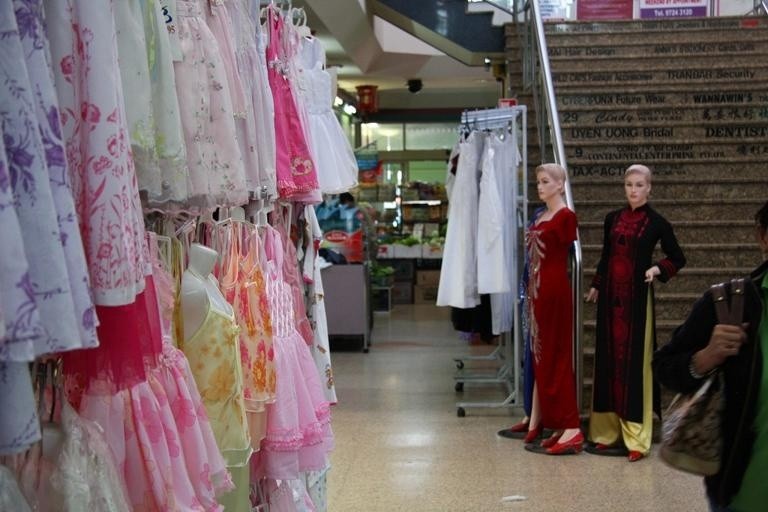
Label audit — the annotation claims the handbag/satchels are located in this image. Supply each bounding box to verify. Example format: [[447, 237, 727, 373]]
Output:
[[660, 367, 727, 475]]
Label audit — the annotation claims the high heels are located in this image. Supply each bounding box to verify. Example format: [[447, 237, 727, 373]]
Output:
[[525, 421, 543, 444], [540, 430, 562, 448], [511, 424, 528, 432], [547, 432, 584, 454]]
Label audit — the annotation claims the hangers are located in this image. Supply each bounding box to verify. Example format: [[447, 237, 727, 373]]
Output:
[[458, 105, 519, 144]]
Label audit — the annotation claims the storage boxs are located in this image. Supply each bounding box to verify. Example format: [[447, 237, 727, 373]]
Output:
[[390, 260, 440, 303]]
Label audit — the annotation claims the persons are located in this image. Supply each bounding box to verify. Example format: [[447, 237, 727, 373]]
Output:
[[180, 241, 251, 512], [585, 163, 687, 463], [650, 202, 768, 511], [510, 206, 546, 444], [521, 161, 585, 454]]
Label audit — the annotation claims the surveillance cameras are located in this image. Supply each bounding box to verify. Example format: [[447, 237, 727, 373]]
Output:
[[407, 79, 422, 93]]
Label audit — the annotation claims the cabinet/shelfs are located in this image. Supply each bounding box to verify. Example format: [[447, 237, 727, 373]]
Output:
[[355, 182, 449, 260]]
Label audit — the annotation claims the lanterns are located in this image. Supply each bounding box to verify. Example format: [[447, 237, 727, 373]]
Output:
[[355, 85, 379, 114]]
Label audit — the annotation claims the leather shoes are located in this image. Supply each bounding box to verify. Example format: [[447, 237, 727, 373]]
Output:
[[629, 451, 642, 461], [595, 443, 607, 450]]
